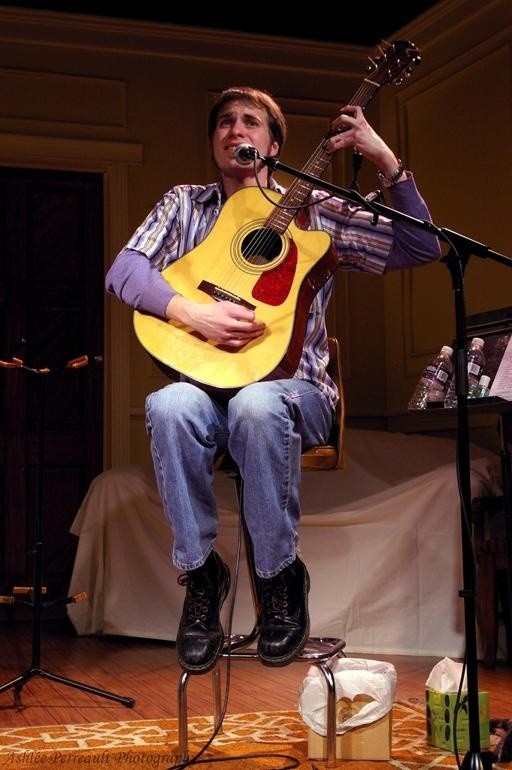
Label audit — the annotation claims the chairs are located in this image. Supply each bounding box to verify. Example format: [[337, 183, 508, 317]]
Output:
[[177, 336, 346, 767]]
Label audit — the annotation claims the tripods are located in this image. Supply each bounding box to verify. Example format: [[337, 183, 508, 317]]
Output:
[[0, 386, 135, 707]]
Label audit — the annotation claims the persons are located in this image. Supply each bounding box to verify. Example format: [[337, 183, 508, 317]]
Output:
[[105, 84, 445, 678]]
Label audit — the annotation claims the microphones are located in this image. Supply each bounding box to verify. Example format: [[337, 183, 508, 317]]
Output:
[[234, 143, 259, 165]]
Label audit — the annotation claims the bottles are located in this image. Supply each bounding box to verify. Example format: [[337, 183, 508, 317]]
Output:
[[405, 337, 491, 413]]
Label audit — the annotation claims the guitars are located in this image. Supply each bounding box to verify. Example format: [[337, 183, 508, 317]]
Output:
[[133, 40, 421, 403]]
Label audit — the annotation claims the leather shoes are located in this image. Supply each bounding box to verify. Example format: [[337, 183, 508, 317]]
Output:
[[176, 552, 230, 675], [258, 555, 310, 668]]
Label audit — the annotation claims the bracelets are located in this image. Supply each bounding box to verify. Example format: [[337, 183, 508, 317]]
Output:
[[376, 157, 406, 190]]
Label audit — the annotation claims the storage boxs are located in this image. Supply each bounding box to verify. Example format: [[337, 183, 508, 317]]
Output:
[[307, 694, 392, 761]]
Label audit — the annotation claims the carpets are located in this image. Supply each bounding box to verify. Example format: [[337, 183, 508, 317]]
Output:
[[0, 696, 511, 770]]
